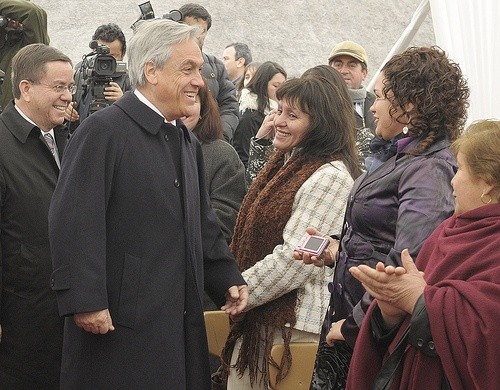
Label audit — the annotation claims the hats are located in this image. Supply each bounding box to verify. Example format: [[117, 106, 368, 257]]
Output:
[[328, 41, 368, 67]]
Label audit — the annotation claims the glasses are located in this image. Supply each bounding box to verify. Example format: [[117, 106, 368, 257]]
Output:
[[27, 78, 78, 95]]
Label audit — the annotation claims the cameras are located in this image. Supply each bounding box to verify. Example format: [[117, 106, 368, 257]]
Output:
[[297, 234, 329, 258]]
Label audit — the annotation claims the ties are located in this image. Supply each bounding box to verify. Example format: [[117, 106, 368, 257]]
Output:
[[44, 132, 61, 171]]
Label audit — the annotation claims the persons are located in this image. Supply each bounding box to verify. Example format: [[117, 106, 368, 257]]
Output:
[[0, 44, 73, 389], [229, 77, 362, 390], [0, 0, 50, 113], [349, 119, 500, 390], [49, 22, 249, 390], [294, 47, 470, 390], [223, 43, 252, 88], [237, 63, 261, 101], [181, 78, 247, 245], [328, 41, 375, 129], [231, 62, 287, 170], [179, 3, 241, 141], [64, 24, 132, 132], [247, 65, 374, 192]]
